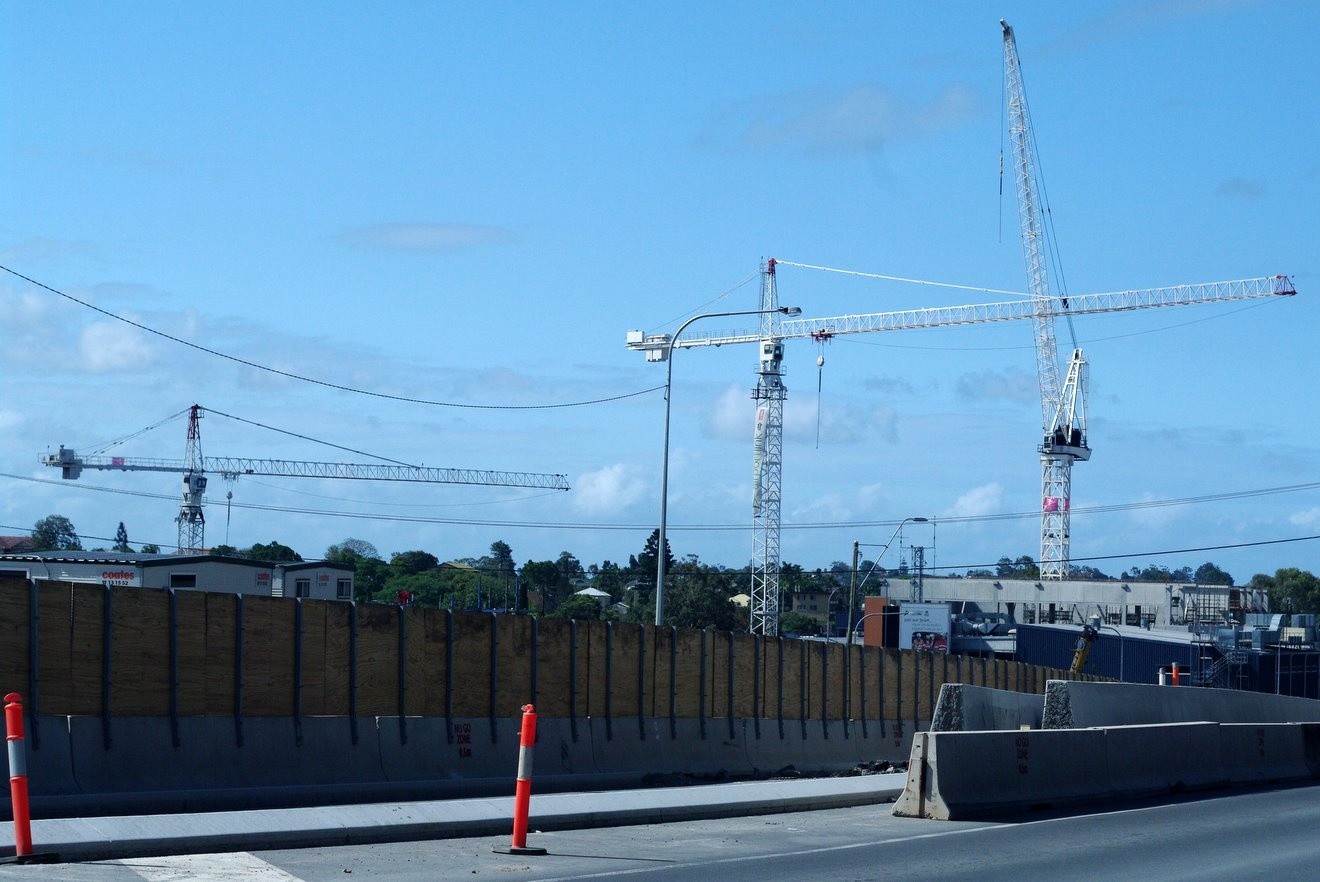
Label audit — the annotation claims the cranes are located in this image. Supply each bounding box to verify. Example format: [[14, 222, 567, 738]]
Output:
[[621, 258, 1302, 636], [34, 402, 574, 559], [990, 15, 1094, 582]]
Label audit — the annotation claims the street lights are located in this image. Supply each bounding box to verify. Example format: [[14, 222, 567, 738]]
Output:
[[844, 516, 928, 643]]
